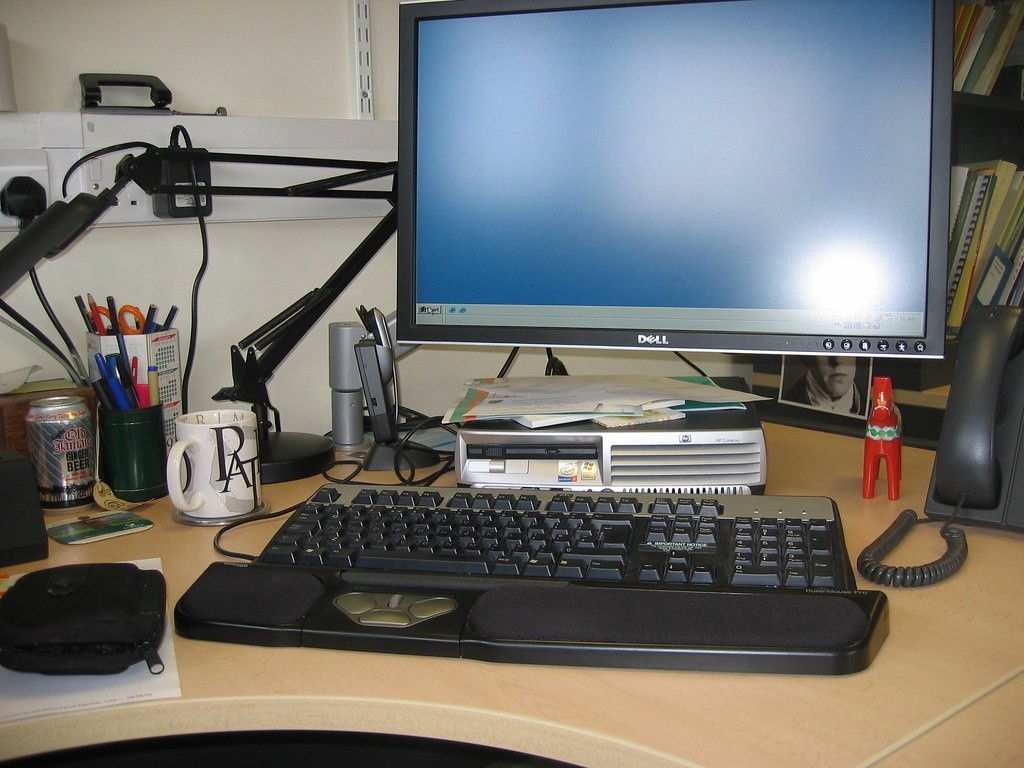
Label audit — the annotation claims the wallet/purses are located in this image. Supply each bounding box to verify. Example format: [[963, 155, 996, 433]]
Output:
[[0, 563, 167, 675]]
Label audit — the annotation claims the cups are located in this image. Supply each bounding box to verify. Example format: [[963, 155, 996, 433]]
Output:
[[166, 410, 260, 519], [98, 401, 167, 501]]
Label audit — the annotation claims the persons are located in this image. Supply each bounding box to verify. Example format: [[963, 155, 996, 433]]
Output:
[[787, 355, 867, 416]]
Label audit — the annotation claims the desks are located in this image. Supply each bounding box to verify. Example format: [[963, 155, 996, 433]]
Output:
[[0, 419, 1024, 768]]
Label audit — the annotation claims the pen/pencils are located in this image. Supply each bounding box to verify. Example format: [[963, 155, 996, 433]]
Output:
[[83, 334, 163, 413], [74, 282, 180, 335]]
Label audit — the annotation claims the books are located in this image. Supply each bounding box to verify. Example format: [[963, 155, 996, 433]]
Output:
[[511, 399, 686, 429], [945, 0, 1024, 338]]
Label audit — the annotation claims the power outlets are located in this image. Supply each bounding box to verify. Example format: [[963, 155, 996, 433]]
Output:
[[79, 148, 207, 221]]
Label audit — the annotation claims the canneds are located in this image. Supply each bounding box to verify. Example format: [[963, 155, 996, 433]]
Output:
[[25, 395, 96, 515]]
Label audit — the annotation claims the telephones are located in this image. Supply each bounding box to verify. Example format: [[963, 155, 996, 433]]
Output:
[[923, 302, 1024, 533]]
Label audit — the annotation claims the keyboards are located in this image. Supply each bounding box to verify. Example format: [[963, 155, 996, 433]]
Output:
[[254, 484, 857, 592]]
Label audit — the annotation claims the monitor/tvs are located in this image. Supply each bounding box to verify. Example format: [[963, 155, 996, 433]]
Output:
[[395, 0, 956, 358]]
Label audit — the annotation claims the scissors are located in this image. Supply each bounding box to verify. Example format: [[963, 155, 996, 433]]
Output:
[[90, 304, 141, 333]]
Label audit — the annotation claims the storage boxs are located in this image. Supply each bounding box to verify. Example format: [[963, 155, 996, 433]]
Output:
[[85, 327, 184, 455], [0, 388, 103, 475], [750, 341, 956, 392]]
